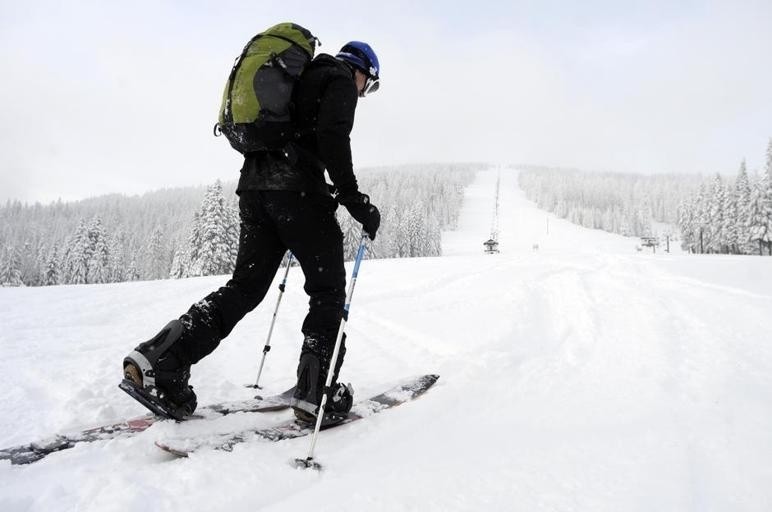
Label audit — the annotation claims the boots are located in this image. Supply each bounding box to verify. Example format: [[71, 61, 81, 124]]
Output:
[[122, 316, 197, 416], [289, 345, 353, 423]]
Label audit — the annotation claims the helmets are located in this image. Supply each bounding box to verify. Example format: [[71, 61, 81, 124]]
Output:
[[336, 40, 380, 79]]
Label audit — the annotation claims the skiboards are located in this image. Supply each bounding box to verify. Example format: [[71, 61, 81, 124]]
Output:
[[0, 375, 439, 465]]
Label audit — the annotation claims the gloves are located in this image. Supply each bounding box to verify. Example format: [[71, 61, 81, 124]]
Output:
[[335, 190, 381, 242]]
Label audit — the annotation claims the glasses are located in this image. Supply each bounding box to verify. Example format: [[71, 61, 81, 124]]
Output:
[[360, 74, 380, 98]]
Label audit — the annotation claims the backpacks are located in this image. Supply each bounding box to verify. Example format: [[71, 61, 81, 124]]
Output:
[[212, 21, 322, 155]]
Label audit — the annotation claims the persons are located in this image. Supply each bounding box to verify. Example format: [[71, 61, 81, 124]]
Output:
[[116, 41, 380, 428]]
